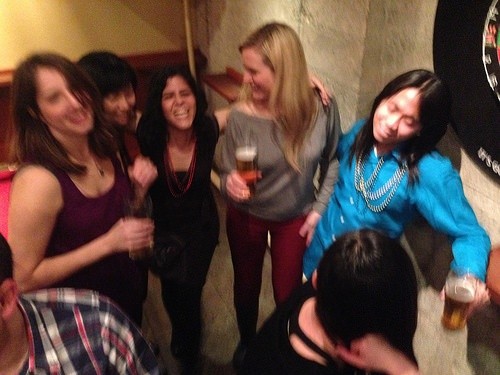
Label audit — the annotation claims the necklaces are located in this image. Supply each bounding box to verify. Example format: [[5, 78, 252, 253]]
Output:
[[354, 145, 408, 212], [79, 158, 105, 178], [163, 136, 198, 198]]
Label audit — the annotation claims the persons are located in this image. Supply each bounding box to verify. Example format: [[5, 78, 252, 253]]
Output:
[[214, 21, 341, 373], [0, 232, 164, 375], [300, 69, 492, 319], [8, 48, 158, 329], [123, 63, 332, 375], [240, 227, 421, 375]]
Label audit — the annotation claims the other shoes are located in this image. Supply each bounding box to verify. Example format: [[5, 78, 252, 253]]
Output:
[[182, 341, 201, 375], [170, 325, 186, 360], [232, 332, 258, 371]]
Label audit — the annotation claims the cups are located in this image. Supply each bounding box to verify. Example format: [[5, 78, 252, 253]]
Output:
[[120, 191, 157, 260], [235, 141, 259, 202], [439, 269, 476, 331]]
